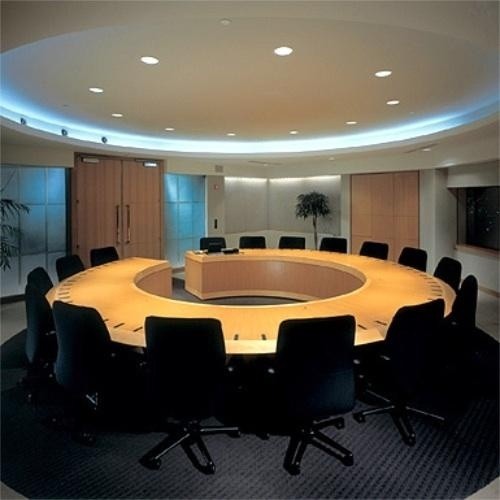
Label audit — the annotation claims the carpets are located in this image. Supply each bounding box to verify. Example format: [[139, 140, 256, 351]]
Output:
[[1, 321, 499, 498]]
